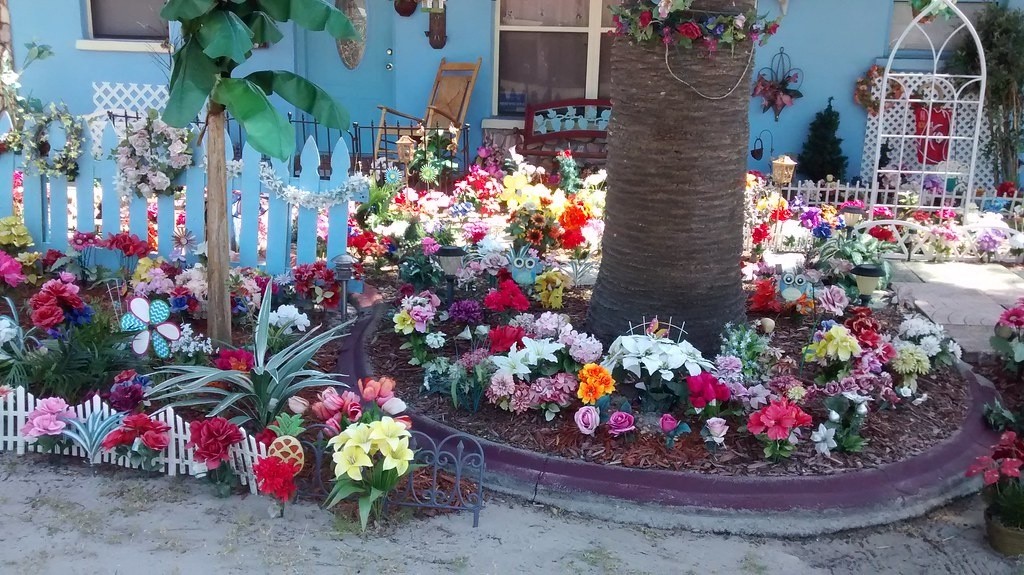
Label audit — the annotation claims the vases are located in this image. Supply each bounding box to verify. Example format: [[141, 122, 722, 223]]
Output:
[[758, 75, 803, 122], [984, 508, 1024, 556], [911, 6, 937, 23]]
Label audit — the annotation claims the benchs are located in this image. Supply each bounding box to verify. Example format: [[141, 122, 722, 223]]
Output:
[[512, 98, 612, 158]]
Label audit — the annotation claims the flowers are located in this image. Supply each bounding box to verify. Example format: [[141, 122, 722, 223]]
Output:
[[853, 66, 900, 116], [608, 1, 782, 49], [908, 0, 958, 20], [0, 89, 25, 155], [22, 100, 87, 181], [111, 117, 194, 200], [754, 71, 799, 113], [964, 430, 1024, 524]]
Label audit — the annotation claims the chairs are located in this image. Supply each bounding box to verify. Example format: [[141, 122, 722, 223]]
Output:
[[371, 58, 484, 192]]
